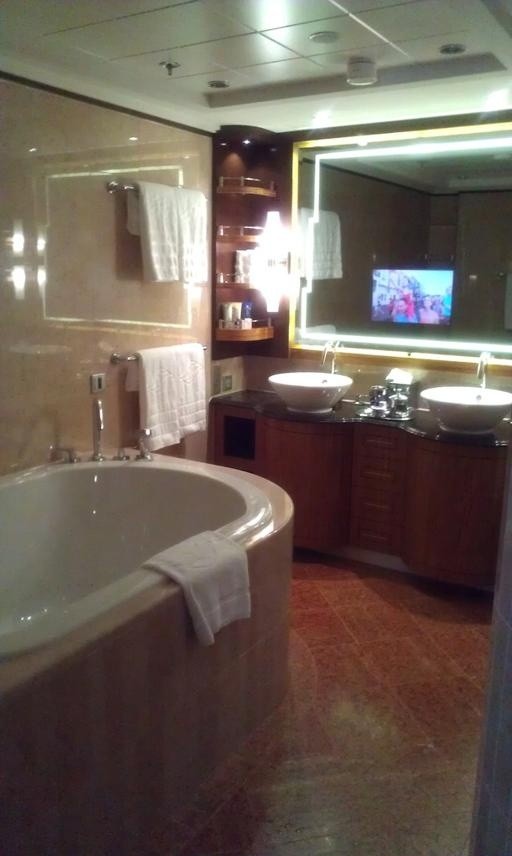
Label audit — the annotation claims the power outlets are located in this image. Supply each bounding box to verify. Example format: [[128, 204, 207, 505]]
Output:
[[222, 374, 233, 391]]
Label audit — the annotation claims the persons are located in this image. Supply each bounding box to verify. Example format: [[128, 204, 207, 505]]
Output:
[[418, 298, 440, 324], [440, 288, 452, 316], [391, 276, 414, 320], [394, 299, 417, 323]]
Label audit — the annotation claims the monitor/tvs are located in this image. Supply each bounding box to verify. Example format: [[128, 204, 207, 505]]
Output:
[[367, 267, 458, 326]]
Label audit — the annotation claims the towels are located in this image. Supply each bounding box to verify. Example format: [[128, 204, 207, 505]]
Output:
[[300, 209, 343, 279], [125, 181, 207, 286], [124, 342, 208, 453], [143, 531, 256, 649]]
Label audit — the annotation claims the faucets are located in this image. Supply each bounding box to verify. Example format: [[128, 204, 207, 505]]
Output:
[[90, 399, 107, 462], [476, 350, 489, 387], [320, 340, 336, 373]]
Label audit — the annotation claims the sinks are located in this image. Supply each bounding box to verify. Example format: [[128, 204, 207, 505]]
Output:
[[420, 385, 512, 434], [268, 372, 353, 413]]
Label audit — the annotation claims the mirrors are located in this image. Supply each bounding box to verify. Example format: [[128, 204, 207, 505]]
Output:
[[289, 138, 511, 358]]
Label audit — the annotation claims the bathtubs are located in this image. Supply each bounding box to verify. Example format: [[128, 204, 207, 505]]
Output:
[[0, 447, 291, 856]]
